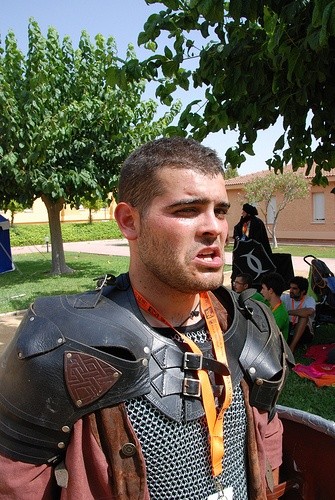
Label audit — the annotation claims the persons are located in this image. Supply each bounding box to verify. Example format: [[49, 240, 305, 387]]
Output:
[[231, 204, 316, 353], [0, 137, 296, 500]]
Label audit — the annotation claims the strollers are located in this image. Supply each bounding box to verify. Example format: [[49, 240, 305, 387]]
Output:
[[303, 254, 335, 329]]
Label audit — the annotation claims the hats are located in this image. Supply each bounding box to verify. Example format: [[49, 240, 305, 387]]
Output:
[[242, 203, 259, 215]]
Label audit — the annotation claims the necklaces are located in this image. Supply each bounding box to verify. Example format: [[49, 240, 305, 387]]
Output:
[[180, 301, 201, 326]]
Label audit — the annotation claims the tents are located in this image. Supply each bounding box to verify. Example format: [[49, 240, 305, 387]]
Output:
[[0, 215, 16, 275]]
